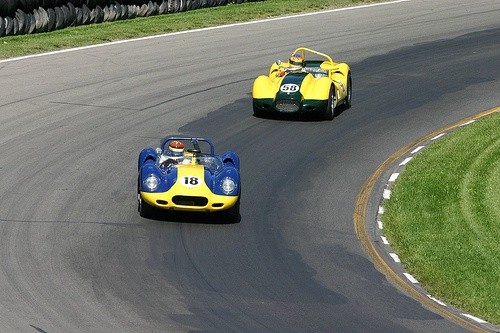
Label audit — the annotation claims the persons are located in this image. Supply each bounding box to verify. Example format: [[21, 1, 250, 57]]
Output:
[[287, 54, 307, 73], [163, 140, 186, 165]]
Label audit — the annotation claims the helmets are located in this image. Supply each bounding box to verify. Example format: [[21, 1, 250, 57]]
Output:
[[168, 141, 184, 156], [288, 54, 304, 68]]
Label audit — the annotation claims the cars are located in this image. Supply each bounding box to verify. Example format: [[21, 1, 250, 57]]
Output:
[[138, 135, 242, 224], [252, 47, 354, 120]]
